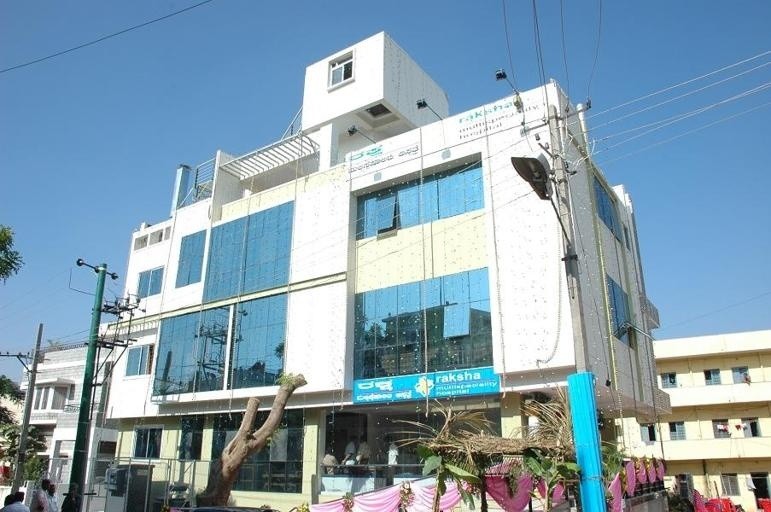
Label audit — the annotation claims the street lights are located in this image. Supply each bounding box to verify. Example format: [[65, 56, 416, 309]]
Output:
[[511, 148, 609, 512]]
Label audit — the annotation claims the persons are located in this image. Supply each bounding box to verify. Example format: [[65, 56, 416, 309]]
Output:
[[340, 433, 358, 465], [0, 492, 30, 512], [387, 438, 399, 467], [323, 449, 340, 474], [47, 484, 61, 512], [356, 435, 371, 463], [62, 481, 82, 512], [742, 372, 752, 385], [30, 478, 51, 512]]
